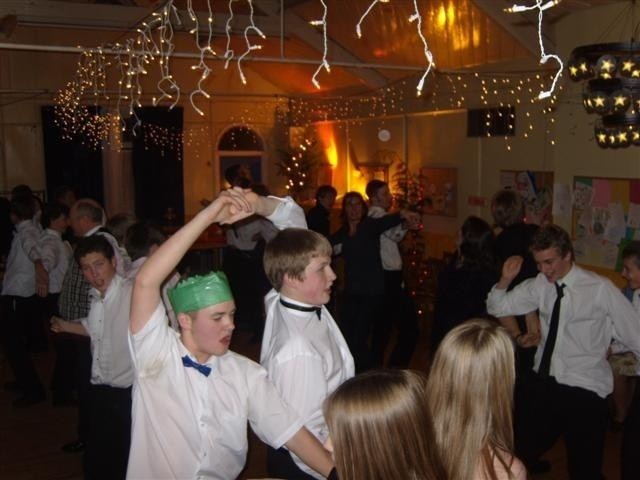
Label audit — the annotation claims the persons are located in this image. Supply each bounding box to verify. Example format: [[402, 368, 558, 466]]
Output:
[[328, 190, 421, 370], [3, 191, 52, 410], [124, 219, 183, 335], [218, 184, 358, 480], [424, 316, 530, 480], [54, 178, 77, 209], [490, 187, 554, 479], [35, 203, 74, 355], [122, 182, 336, 480], [606, 238, 639, 477], [48, 232, 134, 478], [11, 184, 34, 197], [367, 178, 421, 370], [305, 184, 337, 235], [57, 198, 123, 455], [320, 367, 450, 480], [428, 214, 540, 478], [484, 218, 639, 480]]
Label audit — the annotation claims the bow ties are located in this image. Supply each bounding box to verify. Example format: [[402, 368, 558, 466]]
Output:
[[314, 305, 322, 320], [182, 354, 211, 377]]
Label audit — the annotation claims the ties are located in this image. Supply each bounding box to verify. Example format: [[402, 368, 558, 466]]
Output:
[[538, 282, 566, 382]]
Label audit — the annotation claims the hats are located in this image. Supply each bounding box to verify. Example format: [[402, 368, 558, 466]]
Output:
[[167, 269, 237, 314]]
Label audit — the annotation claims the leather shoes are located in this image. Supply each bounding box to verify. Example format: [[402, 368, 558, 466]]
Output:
[[4, 381, 18, 390], [61, 438, 84, 453], [12, 392, 35, 407]]
